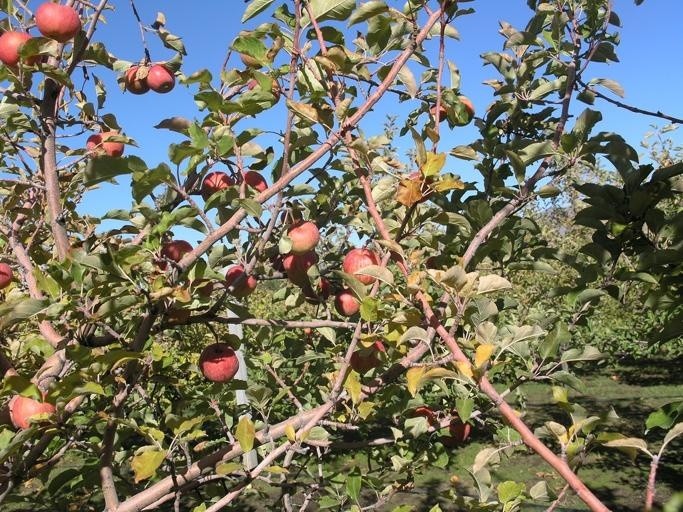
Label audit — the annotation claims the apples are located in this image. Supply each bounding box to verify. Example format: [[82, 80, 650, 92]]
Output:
[[0, 2, 475, 441]]
